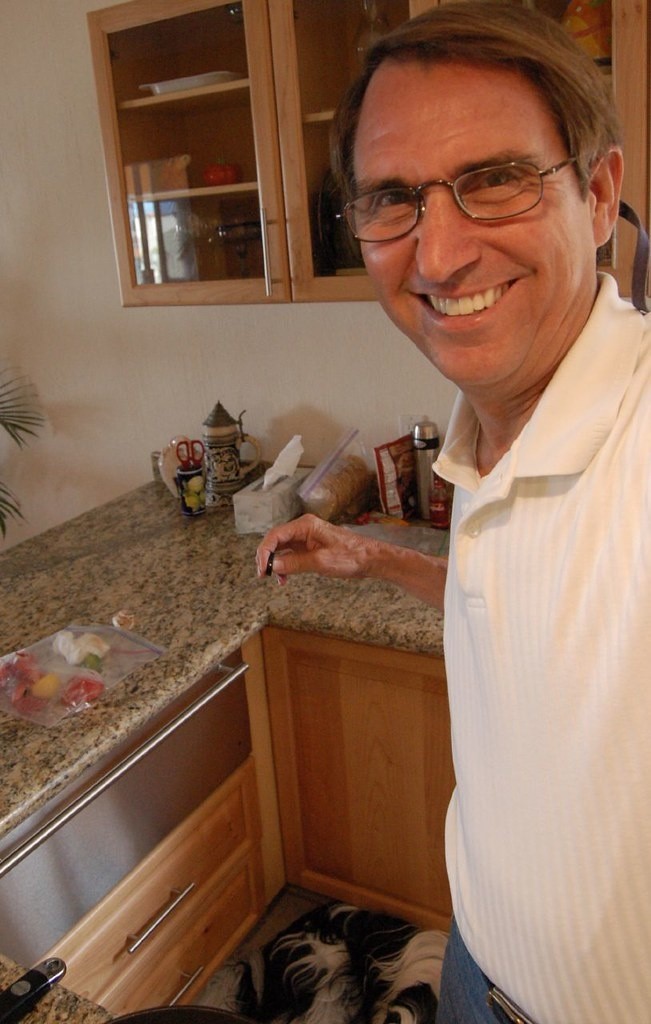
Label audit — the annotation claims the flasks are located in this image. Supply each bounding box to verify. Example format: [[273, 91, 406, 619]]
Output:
[[412, 421, 440, 520]]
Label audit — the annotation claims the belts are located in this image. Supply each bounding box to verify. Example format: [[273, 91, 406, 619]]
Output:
[[478, 969, 528, 1024]]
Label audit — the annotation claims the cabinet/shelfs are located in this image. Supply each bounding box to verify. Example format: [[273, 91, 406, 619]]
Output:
[[85, 0, 651, 308], [28, 755, 266, 1018]]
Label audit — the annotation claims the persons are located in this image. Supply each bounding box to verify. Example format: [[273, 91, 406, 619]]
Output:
[[254, 0, 651, 1024]]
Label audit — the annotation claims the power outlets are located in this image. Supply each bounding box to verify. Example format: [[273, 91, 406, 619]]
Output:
[[398, 413, 427, 437]]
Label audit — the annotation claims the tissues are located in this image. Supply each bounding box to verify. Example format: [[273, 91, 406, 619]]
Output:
[[232, 434, 312, 535]]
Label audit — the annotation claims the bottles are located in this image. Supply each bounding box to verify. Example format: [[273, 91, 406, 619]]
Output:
[[430, 479, 450, 528], [157, 434, 206, 498], [348, 1, 389, 87]]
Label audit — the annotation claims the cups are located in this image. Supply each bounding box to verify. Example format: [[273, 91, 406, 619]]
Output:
[[175, 464, 207, 516], [201, 399, 261, 493]]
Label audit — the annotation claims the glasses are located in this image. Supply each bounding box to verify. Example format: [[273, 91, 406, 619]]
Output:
[[336, 149, 594, 243]]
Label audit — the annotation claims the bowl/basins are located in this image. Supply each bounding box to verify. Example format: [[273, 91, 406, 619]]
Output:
[[96, 1003, 259, 1024]]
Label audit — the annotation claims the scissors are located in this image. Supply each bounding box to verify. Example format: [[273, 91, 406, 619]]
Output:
[[177, 440, 205, 471]]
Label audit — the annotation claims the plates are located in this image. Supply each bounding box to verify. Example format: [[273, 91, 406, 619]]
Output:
[[138, 70, 237, 96]]
